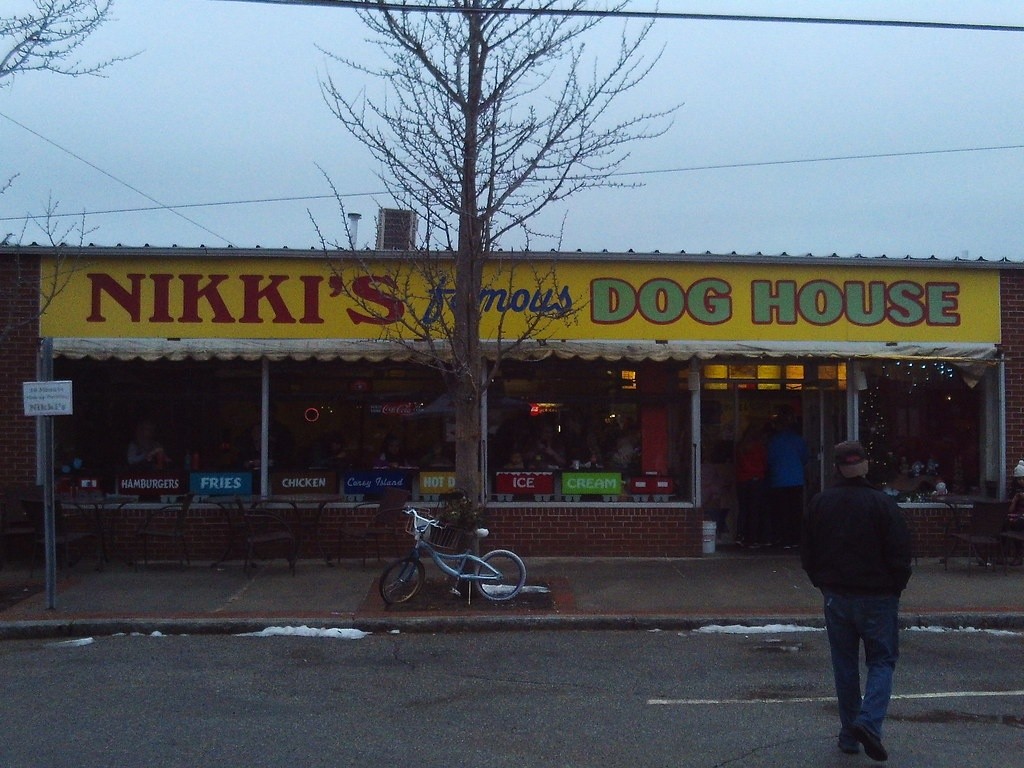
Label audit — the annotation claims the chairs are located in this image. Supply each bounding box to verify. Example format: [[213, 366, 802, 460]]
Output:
[[338, 487, 411, 568], [236, 495, 296, 577], [127, 493, 199, 566], [945, 499, 1016, 577], [899, 506, 917, 566], [21, 498, 95, 569]]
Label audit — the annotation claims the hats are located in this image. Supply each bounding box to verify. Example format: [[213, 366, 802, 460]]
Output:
[[1014, 460, 1024, 477], [833, 441, 865, 465]]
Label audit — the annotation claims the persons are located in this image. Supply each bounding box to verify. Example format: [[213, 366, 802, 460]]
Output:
[[700, 415, 815, 549], [799, 441, 912, 763], [122, 415, 643, 476], [994, 459, 1024, 566]]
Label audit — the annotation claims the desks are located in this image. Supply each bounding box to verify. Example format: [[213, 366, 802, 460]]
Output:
[[941, 497, 986, 566], [273, 493, 349, 567], [203, 495, 272, 569], [82, 497, 141, 571]]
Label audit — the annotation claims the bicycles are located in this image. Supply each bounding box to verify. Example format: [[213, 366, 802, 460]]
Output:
[[378, 509, 526, 607]]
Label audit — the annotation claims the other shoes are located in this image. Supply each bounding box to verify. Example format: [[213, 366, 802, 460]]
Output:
[[784, 544, 791, 549], [839, 743, 858, 753], [736, 535, 745, 548], [793, 544, 799, 547], [850, 724, 887, 761], [748, 543, 759, 548]]
